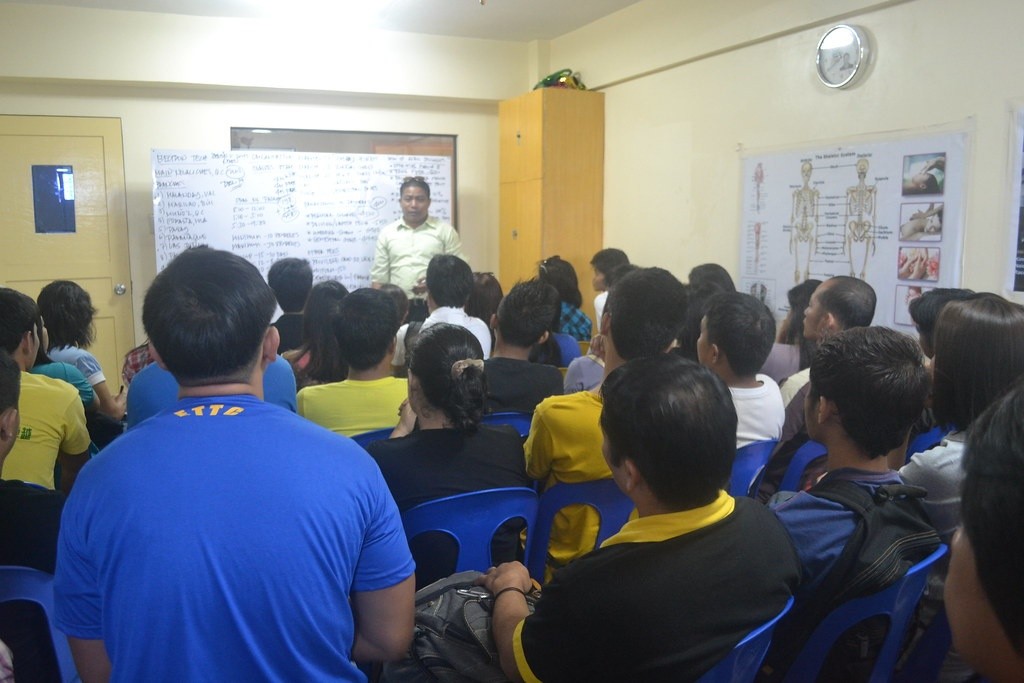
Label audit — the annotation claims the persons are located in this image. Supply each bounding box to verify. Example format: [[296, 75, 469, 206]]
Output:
[[0, 280, 128, 683], [764, 325, 929, 672], [370, 176, 469, 301], [562, 264, 641, 394], [295, 288, 410, 438], [903, 156, 945, 194], [779, 276, 877, 410], [52, 243, 416, 682], [537, 249, 630, 342], [900, 209, 943, 241], [464, 271, 503, 355], [670, 263, 786, 494], [899, 286, 1024, 683], [482, 276, 562, 416], [840, 52, 853, 70], [474, 352, 802, 683], [367, 322, 529, 594], [122, 336, 298, 428], [390, 255, 492, 378], [524, 267, 689, 585], [285, 280, 352, 393], [268, 258, 313, 357]]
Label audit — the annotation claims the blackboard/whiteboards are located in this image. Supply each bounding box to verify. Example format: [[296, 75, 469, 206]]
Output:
[[151, 147, 453, 325]]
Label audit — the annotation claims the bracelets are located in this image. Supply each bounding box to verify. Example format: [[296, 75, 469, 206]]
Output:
[[494, 587, 525, 602]]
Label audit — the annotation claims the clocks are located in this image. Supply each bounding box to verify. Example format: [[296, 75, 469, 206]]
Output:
[[814, 22, 869, 89]]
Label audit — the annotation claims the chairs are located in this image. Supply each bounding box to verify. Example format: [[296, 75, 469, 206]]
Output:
[[0, 410, 988, 683]]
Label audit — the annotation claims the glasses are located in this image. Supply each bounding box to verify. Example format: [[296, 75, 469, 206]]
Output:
[[402, 175, 425, 184], [538, 255, 561, 280]]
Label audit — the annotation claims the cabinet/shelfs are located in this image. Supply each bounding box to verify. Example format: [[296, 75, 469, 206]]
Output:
[[496, 87, 605, 335]]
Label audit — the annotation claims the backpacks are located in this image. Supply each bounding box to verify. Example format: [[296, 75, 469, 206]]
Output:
[[382, 569, 549, 683], [765, 477, 942, 683]]
